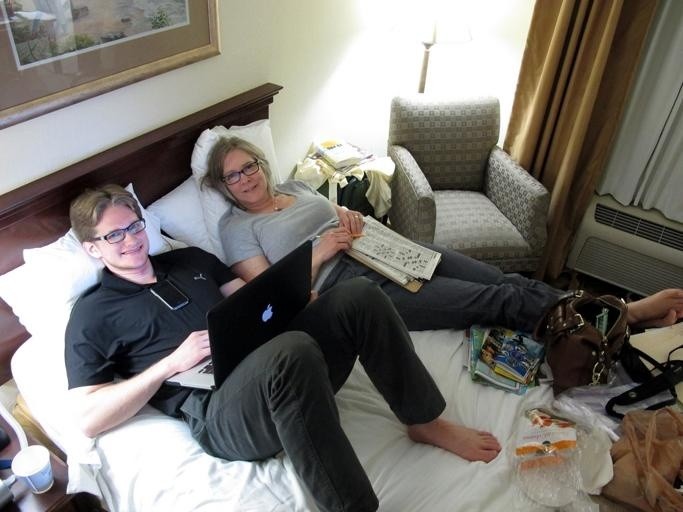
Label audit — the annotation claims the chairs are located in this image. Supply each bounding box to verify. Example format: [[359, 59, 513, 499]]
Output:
[[387, 95, 552, 281]]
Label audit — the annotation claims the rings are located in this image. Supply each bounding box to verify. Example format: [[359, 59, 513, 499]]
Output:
[[354, 215, 359, 218]]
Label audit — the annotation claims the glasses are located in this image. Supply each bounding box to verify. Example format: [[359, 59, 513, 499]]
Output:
[[220, 158, 259, 185], [92, 218, 146, 244]]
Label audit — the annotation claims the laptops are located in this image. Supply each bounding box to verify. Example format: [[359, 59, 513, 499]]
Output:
[[164, 239, 312, 391]]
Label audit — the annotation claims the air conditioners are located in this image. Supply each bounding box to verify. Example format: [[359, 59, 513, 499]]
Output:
[[565, 191, 683, 298]]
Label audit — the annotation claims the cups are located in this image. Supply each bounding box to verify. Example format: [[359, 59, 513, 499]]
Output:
[[10, 445, 54, 495]]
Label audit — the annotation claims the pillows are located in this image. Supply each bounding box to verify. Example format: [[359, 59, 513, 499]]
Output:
[[190, 118, 285, 266], [1, 211, 163, 354], [22, 183, 187, 261], [147, 173, 216, 257]]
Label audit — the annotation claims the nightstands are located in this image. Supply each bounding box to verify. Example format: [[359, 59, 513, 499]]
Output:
[[0, 415, 77, 512]]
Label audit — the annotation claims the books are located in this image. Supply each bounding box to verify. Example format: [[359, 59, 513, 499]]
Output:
[[344, 215, 442, 293], [308, 138, 375, 174], [461, 322, 545, 395]]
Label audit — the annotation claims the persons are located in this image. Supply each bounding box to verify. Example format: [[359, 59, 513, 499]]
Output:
[[198, 135, 683, 330], [64, 184, 501, 512]]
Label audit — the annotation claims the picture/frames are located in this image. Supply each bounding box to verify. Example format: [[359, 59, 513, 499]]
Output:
[[0, 0, 220, 127]]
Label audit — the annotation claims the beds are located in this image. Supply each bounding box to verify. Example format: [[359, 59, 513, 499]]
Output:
[[1, 84, 682, 512]]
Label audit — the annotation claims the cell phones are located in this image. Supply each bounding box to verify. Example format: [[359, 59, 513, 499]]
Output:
[[149, 278, 190, 312]]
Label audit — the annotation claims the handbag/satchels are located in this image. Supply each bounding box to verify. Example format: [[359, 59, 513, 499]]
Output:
[[532, 288, 629, 396]]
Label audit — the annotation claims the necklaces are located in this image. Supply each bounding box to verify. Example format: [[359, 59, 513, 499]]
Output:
[[269, 197, 283, 212]]
